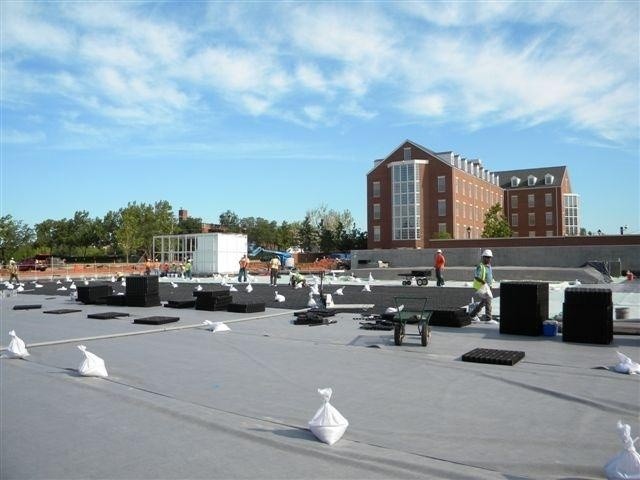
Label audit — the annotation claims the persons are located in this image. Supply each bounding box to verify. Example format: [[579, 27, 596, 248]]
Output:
[[8, 261, 20, 284], [289, 266, 307, 287], [115, 271, 124, 281], [9, 257, 14, 265], [269, 255, 281, 286], [434, 250, 445, 286], [145, 258, 193, 280], [238, 253, 249, 282], [469, 249, 496, 320]]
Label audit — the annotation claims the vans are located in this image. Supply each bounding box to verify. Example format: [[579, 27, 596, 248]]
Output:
[[17, 254, 49, 271]]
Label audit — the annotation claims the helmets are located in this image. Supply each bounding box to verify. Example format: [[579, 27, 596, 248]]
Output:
[[481, 250, 493, 257], [437, 249, 442, 253]]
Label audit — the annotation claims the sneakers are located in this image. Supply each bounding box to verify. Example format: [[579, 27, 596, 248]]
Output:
[[470, 315, 480, 321], [485, 320, 497, 324]]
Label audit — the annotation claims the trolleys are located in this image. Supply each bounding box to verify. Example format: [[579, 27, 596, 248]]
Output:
[[398, 268, 434, 285], [392, 295, 433, 346]]
